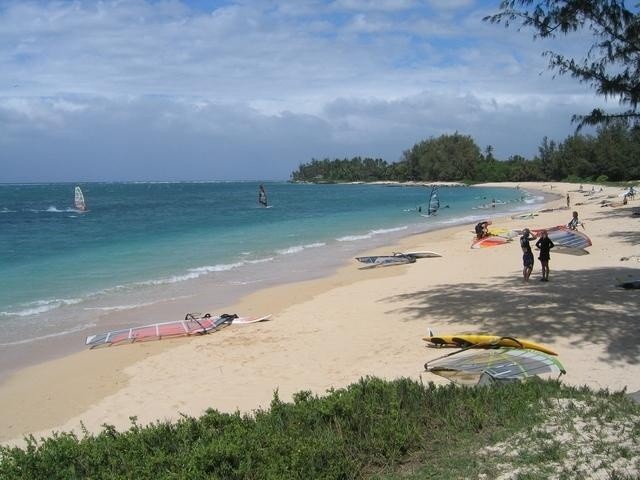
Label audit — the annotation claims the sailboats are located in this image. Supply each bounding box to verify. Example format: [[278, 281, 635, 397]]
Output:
[[532, 224, 593, 250], [85, 314, 273, 349], [468, 228, 526, 250], [355, 251, 442, 268], [74, 186, 93, 213], [422, 334, 567, 389], [417, 185, 441, 218], [257, 183, 273, 209]]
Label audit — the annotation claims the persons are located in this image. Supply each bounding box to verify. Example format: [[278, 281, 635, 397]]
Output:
[[535, 229, 555, 281], [567, 211, 586, 231], [565, 183, 639, 208], [475, 221, 492, 240], [520, 228, 537, 282]]
